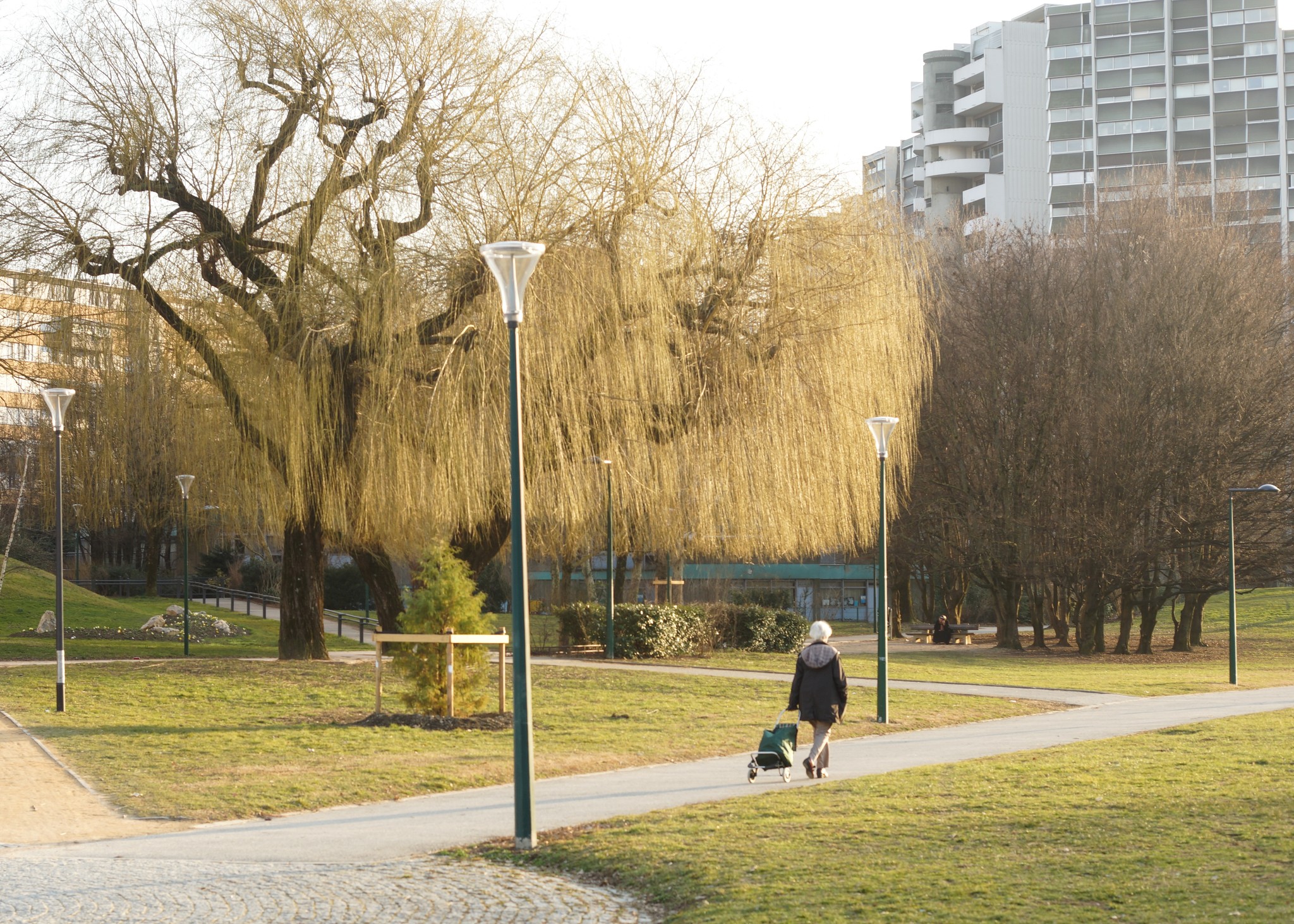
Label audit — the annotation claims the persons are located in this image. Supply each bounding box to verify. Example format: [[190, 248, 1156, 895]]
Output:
[[933, 615, 952, 645], [787, 620, 848, 779]]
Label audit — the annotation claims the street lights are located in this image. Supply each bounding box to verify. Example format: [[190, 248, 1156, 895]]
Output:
[[1226, 483, 1281, 684], [864, 417, 902, 724], [34, 386, 81, 714], [472, 239, 550, 853], [172, 473, 196, 660]]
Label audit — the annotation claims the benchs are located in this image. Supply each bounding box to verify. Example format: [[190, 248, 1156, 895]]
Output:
[[905, 624, 979, 645]]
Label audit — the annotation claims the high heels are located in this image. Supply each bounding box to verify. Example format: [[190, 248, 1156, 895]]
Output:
[[817, 768, 828, 778], [802, 756, 817, 779]]
